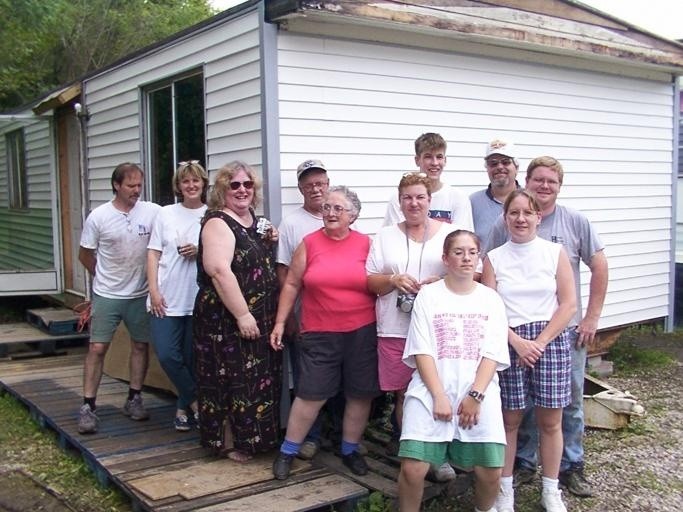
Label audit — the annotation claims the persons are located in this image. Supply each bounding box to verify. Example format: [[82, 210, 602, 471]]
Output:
[[364, 173, 483, 482], [78, 163, 164, 433], [191, 161, 281, 462], [480, 188, 578, 511], [146, 160, 212, 433], [268, 185, 373, 480], [514, 156, 609, 496], [397, 230, 511, 512], [385, 132, 476, 232], [469, 138, 524, 257], [275, 158, 356, 461]]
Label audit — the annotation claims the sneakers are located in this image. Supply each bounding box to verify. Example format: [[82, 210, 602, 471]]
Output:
[[433, 460, 460, 482], [512, 463, 540, 488], [76, 403, 102, 434], [538, 486, 568, 511], [559, 460, 596, 498], [297, 436, 324, 459], [122, 395, 151, 421], [493, 490, 516, 511], [334, 438, 371, 477], [172, 410, 200, 431], [270, 450, 297, 480]]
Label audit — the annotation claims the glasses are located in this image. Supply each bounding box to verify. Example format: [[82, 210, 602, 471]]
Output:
[[401, 172, 430, 179], [319, 204, 353, 218], [485, 156, 512, 169], [227, 181, 254, 191]]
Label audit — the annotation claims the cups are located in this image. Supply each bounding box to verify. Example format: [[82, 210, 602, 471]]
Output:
[[174, 233, 188, 255]]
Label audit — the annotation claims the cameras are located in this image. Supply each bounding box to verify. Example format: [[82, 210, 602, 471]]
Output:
[[396, 293, 416, 313]]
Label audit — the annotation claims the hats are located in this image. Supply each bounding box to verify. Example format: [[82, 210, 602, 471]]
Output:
[[482, 136, 519, 166], [296, 158, 328, 182]]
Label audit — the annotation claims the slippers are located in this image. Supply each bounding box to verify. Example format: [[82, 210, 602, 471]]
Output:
[[222, 444, 254, 463]]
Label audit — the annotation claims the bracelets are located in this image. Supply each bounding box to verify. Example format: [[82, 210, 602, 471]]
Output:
[[389, 272, 398, 289]]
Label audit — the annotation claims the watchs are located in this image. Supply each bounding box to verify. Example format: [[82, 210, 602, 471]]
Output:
[[468, 391, 485, 403]]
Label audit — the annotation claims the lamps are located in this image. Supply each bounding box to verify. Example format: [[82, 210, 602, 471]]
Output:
[[73, 102, 93, 122]]
